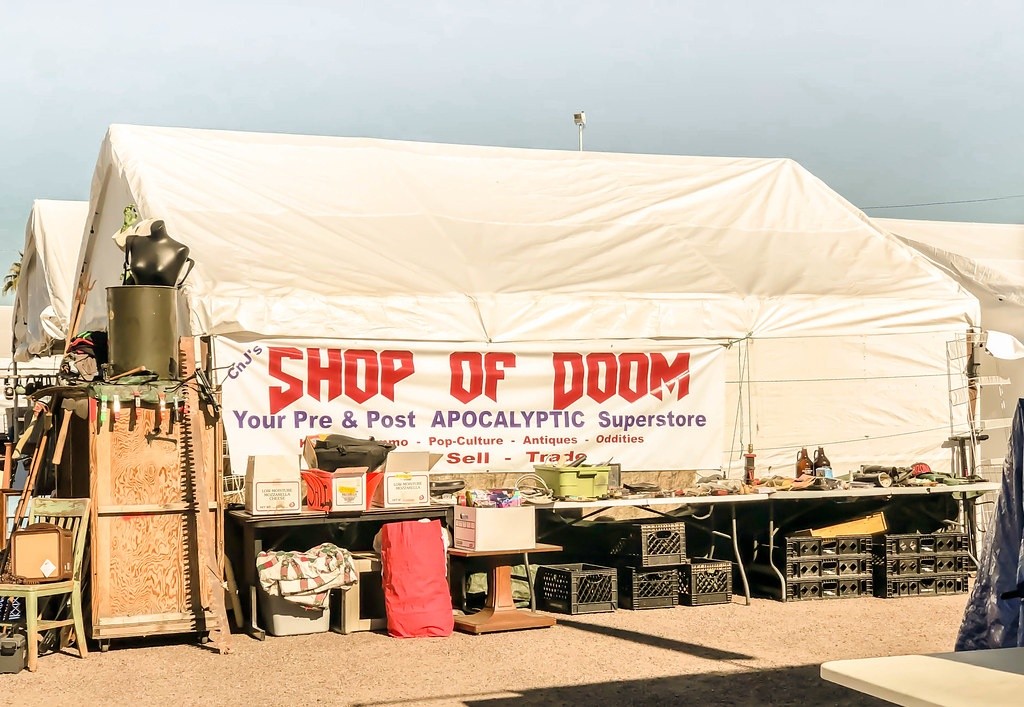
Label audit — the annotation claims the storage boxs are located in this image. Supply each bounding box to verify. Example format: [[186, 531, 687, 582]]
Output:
[[244, 452, 444, 515], [542, 523, 732, 615], [753, 512, 970, 603], [535, 465, 611, 501], [258, 588, 329, 637], [453, 505, 536, 551]]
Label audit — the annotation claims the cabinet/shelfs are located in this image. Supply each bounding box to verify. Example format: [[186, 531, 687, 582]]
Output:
[[54, 385, 230, 651]]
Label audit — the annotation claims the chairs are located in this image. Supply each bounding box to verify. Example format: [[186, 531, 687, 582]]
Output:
[[0, 498, 90, 672]]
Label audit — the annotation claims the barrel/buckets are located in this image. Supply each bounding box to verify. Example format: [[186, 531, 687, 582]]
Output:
[[105, 286, 178, 380]]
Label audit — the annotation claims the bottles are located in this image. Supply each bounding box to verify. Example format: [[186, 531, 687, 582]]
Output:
[[812, 446, 831, 476], [796, 447, 813, 478]]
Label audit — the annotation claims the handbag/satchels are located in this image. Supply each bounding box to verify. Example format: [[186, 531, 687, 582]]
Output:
[[314, 433, 394, 472]]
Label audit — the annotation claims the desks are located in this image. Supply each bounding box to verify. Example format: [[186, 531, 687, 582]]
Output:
[[520, 493, 770, 613], [770, 478, 1003, 602], [447, 543, 563, 634], [226, 508, 536, 641], [821, 647, 1024, 706]]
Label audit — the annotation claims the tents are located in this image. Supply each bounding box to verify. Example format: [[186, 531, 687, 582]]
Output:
[[11, 197, 91, 361], [66, 120, 983, 566], [866, 217, 1024, 548]]
